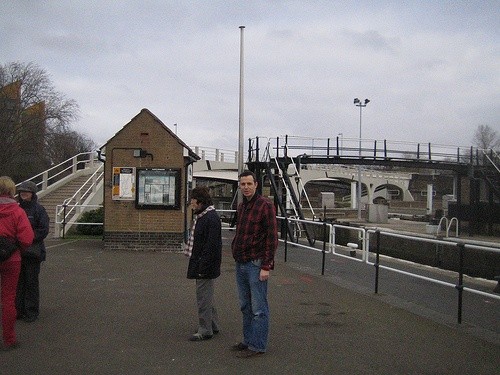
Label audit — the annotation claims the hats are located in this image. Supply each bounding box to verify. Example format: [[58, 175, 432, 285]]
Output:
[[17, 181, 36, 192]]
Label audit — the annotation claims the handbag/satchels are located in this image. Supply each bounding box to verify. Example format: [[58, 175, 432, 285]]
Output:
[[0, 236, 17, 261], [21, 240, 40, 258]]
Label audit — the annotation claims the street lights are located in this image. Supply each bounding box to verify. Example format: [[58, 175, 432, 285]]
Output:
[[174, 123, 177, 134], [353, 97, 371, 221]]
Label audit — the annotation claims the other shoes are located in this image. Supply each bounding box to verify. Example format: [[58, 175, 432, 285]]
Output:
[[15, 311, 23, 319], [24, 314, 36, 322], [233, 342, 248, 350], [236, 348, 264, 358], [195, 327, 219, 333], [192, 333, 213, 341], [4, 344, 16, 351]]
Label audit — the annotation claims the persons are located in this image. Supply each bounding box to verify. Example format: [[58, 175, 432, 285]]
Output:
[[0, 176, 34, 351], [16, 181, 50, 323], [186, 187, 222, 341], [231, 171, 278, 357]]
